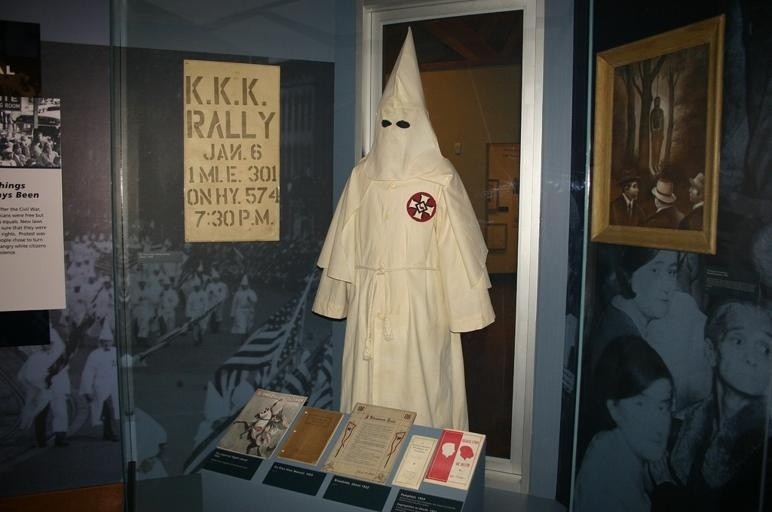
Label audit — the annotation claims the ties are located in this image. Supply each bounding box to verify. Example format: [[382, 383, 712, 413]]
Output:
[[628, 202, 631, 218]]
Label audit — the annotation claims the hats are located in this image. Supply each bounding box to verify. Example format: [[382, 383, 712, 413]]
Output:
[[651, 177, 677, 204], [204, 381, 233, 424], [240, 273, 249, 286], [135, 406, 168, 471], [71, 235, 220, 287], [688, 172, 705, 194], [98, 315, 115, 341], [615, 168, 641, 185]]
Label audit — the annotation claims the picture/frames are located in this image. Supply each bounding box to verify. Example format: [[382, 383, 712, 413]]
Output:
[[592, 14, 725, 257], [487, 180, 502, 211], [484, 222, 509, 252]]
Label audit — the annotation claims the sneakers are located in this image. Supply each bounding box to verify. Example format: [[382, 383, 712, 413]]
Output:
[[39, 432, 70, 448], [103, 432, 120, 442]]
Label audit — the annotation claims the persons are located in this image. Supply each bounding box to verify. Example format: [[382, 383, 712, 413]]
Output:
[[639, 178, 684, 230], [610, 169, 647, 226], [649, 97, 664, 175], [577, 242, 681, 461], [19, 230, 261, 449], [649, 288, 771, 511], [678, 172, 705, 231], [574, 332, 676, 509], [0, 123, 62, 169]]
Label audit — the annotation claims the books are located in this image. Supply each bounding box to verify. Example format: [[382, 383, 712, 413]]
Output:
[[328, 399, 417, 486], [278, 404, 345, 470], [220, 386, 308, 460], [425, 427, 489, 490]]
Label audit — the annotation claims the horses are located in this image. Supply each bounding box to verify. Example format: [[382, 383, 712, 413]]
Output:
[[232, 407, 272, 457]]
